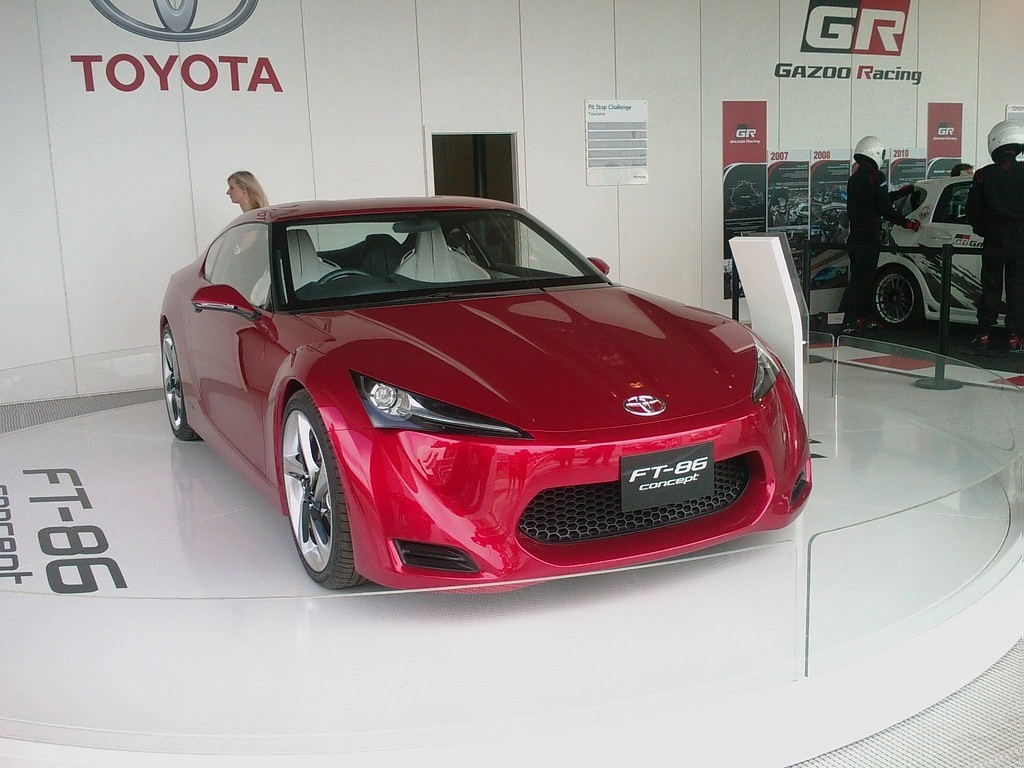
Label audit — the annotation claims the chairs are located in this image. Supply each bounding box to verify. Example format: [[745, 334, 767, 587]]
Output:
[[246, 228, 344, 305], [345, 233, 415, 277], [396, 223, 492, 285]]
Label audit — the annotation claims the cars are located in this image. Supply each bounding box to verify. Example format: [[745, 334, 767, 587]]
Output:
[[870, 177, 1006, 328], [160, 197, 811, 596], [811, 267, 850, 287]]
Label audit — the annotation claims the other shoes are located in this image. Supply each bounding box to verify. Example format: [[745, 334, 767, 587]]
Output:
[[866, 319, 884, 331], [970, 333, 989, 349], [841, 320, 866, 338], [1007, 334, 1024, 352]]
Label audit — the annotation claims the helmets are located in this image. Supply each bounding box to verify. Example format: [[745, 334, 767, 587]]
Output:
[[854, 136, 886, 171], [987, 120, 1023, 162]]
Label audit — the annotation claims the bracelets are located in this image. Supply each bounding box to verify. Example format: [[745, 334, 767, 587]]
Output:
[[233, 243, 244, 256]]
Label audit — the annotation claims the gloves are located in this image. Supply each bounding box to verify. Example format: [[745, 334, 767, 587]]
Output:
[[905, 219, 921, 233], [899, 184, 914, 198]]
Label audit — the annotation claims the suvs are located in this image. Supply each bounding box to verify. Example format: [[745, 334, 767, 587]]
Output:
[[789, 202, 808, 225], [822, 202, 847, 214]]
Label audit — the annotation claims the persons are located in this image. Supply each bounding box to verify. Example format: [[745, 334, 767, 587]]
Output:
[[217, 171, 270, 272], [950, 163, 973, 177], [838, 137, 921, 332], [965, 120, 1024, 353]]
